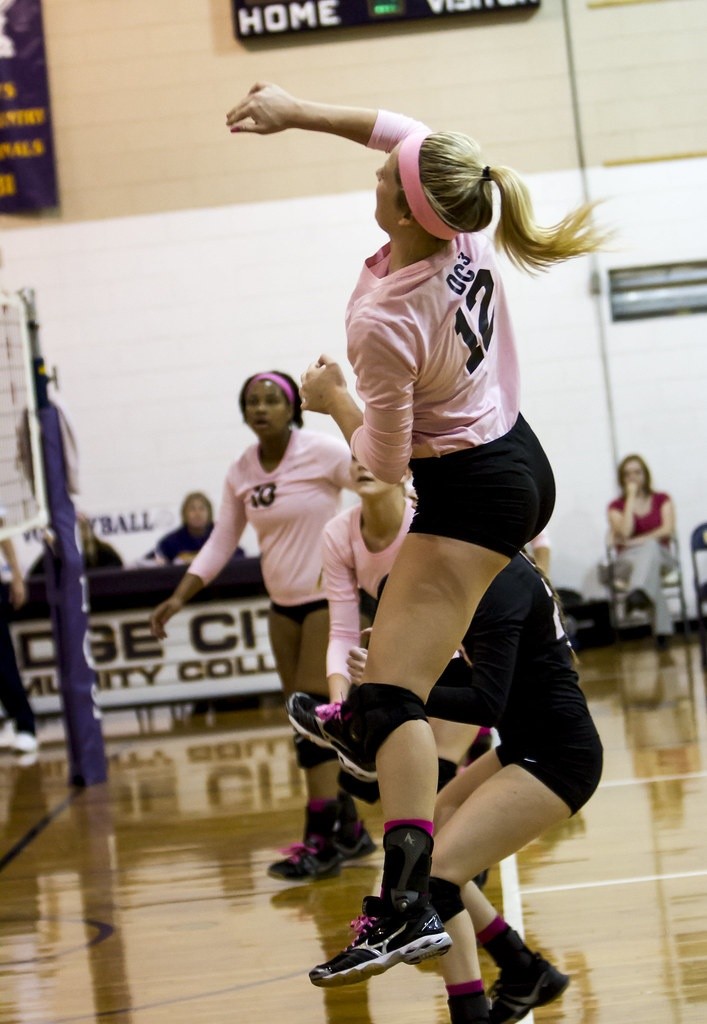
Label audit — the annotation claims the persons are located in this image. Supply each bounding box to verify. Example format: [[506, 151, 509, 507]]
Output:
[[135, 83, 603, 1024], [24, 512, 124, 578], [606, 455, 674, 646], [0, 506, 39, 752]]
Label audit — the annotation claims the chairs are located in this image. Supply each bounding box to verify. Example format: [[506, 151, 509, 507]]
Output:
[[691, 523, 707, 631], [606, 535, 689, 650]]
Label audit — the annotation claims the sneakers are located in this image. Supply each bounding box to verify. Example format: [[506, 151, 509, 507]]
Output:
[[310, 893, 453, 988], [287, 691, 377, 783], [332, 820, 376, 860], [268, 831, 344, 882], [488, 952, 569, 1024]]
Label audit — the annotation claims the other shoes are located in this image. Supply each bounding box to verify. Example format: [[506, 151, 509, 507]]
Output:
[[627, 592, 648, 610], [11, 733, 39, 753], [655, 636, 670, 651]]
